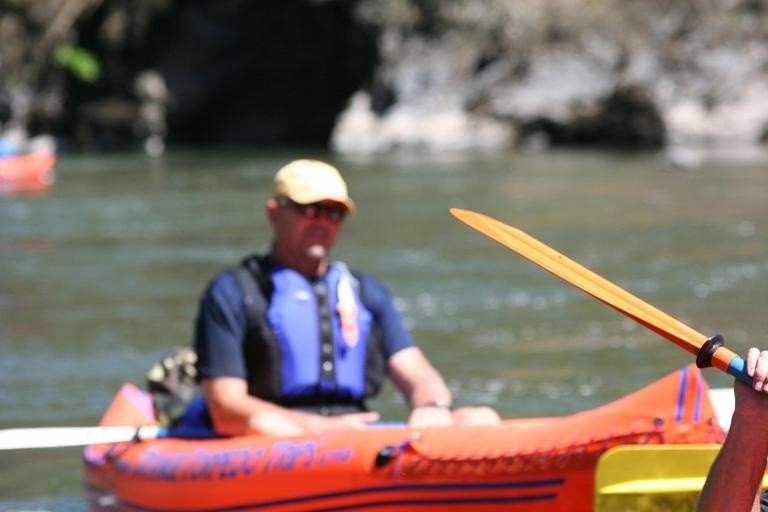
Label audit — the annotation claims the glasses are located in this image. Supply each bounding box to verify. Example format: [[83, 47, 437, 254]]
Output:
[[277, 201, 345, 223]]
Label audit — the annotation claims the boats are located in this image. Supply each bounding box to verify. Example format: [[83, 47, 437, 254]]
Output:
[[1, 151, 56, 192], [83, 363, 761, 512]]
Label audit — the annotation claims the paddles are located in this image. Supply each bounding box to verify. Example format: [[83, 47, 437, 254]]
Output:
[[0, 418, 408, 450], [593, 444, 768, 512], [449, 207, 768, 394]]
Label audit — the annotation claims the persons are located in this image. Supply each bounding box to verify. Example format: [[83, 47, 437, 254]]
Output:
[[696, 348, 768, 512], [195, 158, 501, 436]]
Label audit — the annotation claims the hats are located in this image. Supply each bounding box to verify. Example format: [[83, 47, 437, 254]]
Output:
[[270, 159, 352, 213]]
